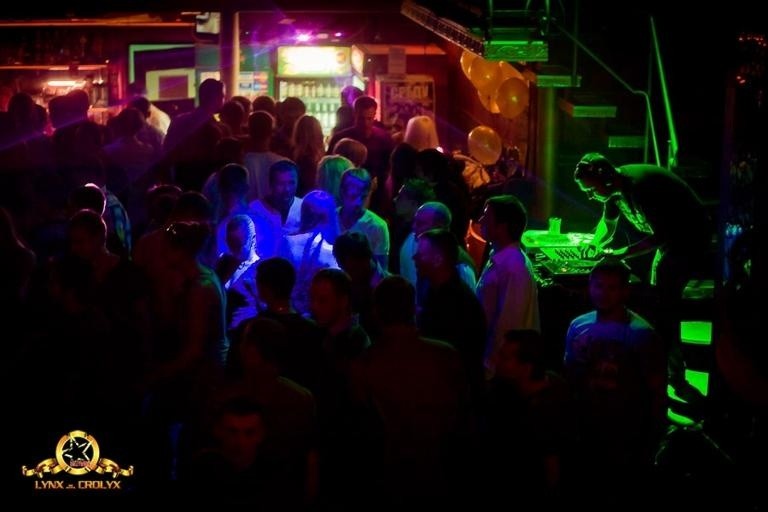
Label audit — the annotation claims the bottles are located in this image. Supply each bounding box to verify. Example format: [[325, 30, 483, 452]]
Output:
[[279, 79, 348, 129], [83, 71, 106, 108], [2, 29, 102, 64]]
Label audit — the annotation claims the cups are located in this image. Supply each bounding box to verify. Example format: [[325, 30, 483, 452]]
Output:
[[548, 216, 562, 237]]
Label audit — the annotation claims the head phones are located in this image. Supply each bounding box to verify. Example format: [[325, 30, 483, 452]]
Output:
[[577, 158, 617, 194]]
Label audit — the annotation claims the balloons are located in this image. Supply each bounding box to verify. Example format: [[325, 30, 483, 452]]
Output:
[[458, 49, 528, 167]]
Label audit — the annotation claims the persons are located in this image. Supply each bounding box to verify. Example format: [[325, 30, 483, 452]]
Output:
[[0, 76, 715, 511]]
